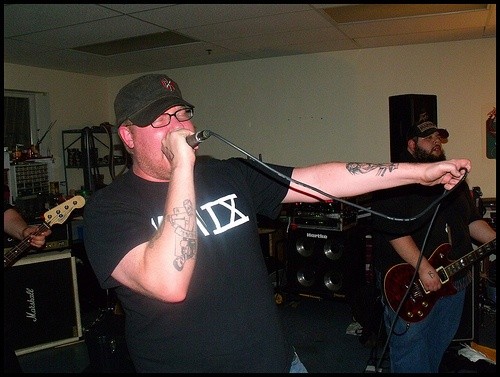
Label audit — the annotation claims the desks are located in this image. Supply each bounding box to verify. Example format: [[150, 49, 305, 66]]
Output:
[[257, 228, 275, 256]]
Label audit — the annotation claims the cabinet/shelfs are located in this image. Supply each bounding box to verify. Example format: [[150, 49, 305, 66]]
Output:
[[62, 129, 128, 241], [9, 162, 51, 201]]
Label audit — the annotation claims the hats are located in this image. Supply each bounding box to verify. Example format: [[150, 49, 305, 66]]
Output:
[[409, 120, 449, 139], [114, 74, 195, 129]]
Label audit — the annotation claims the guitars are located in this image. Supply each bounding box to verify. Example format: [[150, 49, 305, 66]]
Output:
[[0, 195, 87, 268], [383, 233, 496, 325]]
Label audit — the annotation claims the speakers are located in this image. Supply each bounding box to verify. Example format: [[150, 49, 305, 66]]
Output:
[[389, 94, 437, 163], [283, 227, 365, 305]]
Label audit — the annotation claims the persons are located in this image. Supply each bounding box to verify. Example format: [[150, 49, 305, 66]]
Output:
[[82, 76, 472, 373], [3, 184, 52, 374], [372, 121, 496, 373]]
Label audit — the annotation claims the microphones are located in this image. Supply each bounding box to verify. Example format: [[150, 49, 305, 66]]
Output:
[[185, 129, 212, 149]]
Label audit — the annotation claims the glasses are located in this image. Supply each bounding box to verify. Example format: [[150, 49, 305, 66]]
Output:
[[124, 107, 194, 128]]
